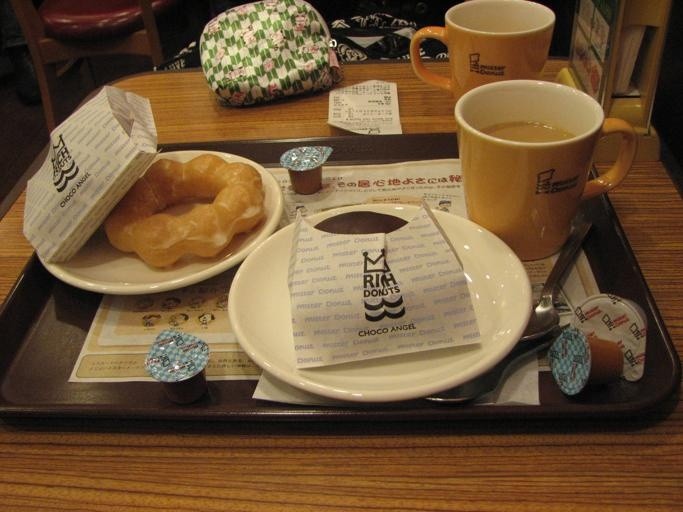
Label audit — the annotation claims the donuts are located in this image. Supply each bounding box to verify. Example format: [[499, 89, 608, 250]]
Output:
[[103, 154, 263, 268]]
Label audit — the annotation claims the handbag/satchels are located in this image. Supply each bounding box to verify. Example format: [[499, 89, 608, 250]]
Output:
[[199, 0, 342, 107]]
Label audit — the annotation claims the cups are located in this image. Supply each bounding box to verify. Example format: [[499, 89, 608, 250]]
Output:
[[617, 295, 649, 331], [157, 367, 207, 404], [452, 80, 636, 259], [582, 333, 624, 397], [288, 164, 324, 195], [409, 0, 555, 95]]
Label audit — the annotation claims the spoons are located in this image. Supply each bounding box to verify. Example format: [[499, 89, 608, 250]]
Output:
[[517, 209, 597, 340]]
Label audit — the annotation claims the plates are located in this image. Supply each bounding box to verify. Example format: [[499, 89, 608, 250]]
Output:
[[33, 149, 282, 296], [227, 202, 533, 403]]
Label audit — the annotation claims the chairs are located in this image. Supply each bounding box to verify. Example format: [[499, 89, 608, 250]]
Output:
[[10, 0, 177, 141]]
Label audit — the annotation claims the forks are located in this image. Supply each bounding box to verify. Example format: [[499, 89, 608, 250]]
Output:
[[425, 321, 572, 404]]
